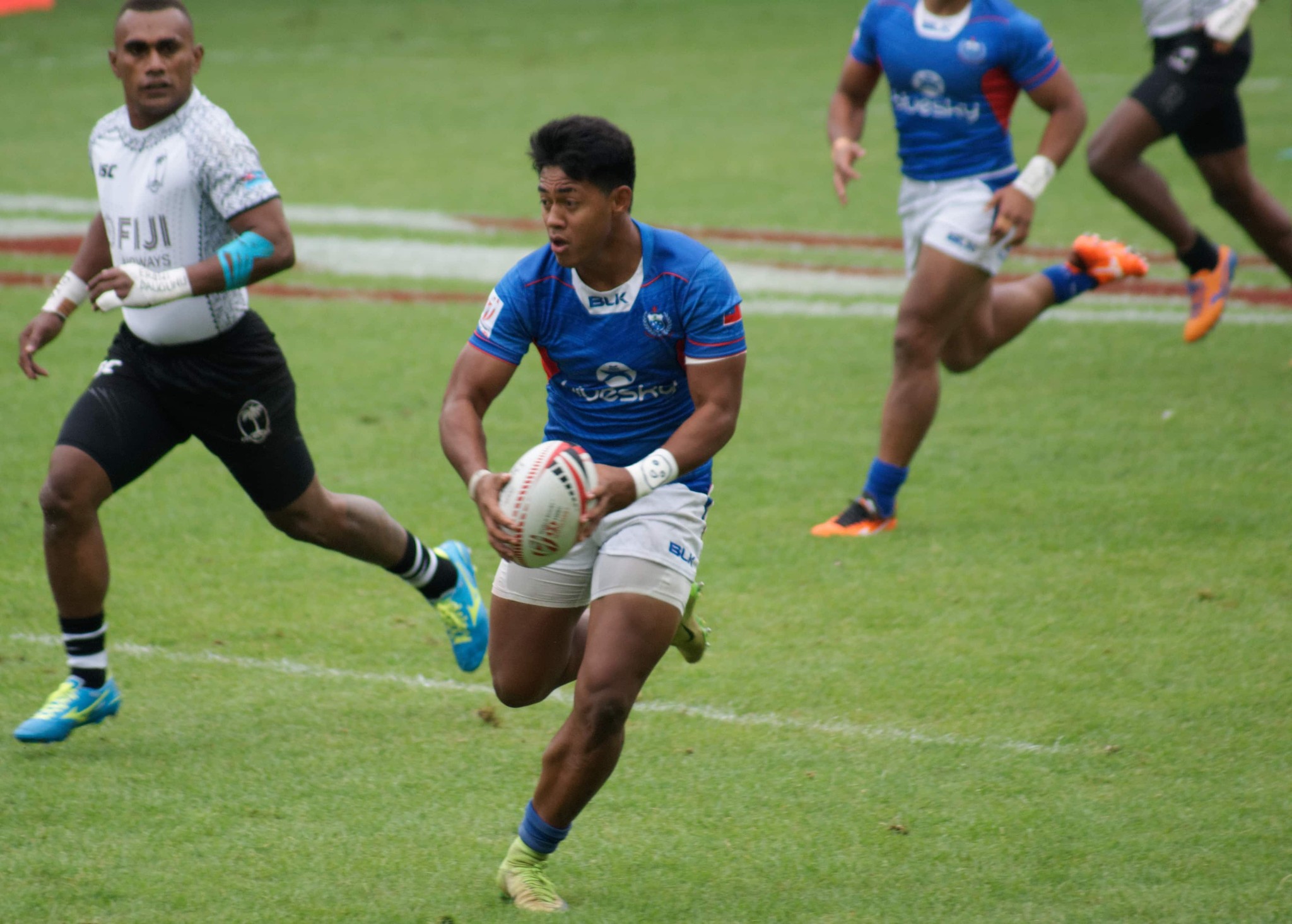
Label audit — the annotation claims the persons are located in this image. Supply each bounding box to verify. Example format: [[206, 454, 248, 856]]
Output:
[[12, 0, 488, 742], [441, 116, 748, 914], [803, 0, 1152, 538], [1084, 0, 1292, 342]]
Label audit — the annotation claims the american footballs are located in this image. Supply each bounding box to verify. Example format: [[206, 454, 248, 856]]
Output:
[[496, 439, 599, 570]]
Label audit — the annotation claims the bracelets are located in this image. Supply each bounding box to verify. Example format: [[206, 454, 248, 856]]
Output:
[[469, 470, 490, 500]]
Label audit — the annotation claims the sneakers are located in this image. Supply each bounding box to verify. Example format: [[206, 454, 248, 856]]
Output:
[[1184, 245, 1237, 342], [670, 580, 711, 663], [809, 491, 897, 536], [427, 540, 489, 672], [495, 838, 567, 916], [1067, 233, 1148, 284], [13, 676, 120, 742]]
